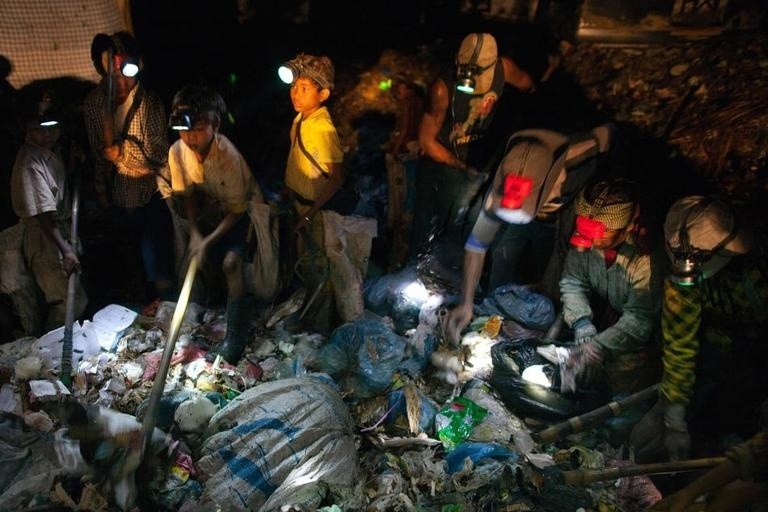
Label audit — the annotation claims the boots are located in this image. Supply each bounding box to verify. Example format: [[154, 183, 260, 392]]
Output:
[[205, 295, 250, 366]]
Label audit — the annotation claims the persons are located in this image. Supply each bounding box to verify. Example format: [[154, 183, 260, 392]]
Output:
[[83, 31, 169, 326], [0, 94, 91, 339], [281, 26, 610, 347], [559, 181, 667, 447], [630, 194, 768, 488], [172, 88, 265, 364]]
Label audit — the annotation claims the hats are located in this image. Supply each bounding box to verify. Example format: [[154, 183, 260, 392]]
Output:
[[453, 33, 498, 97], [663, 195, 756, 287], [487, 127, 573, 225], [16, 86, 77, 128]]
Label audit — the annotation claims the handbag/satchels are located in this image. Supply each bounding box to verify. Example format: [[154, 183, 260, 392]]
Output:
[[328, 161, 364, 216]]
